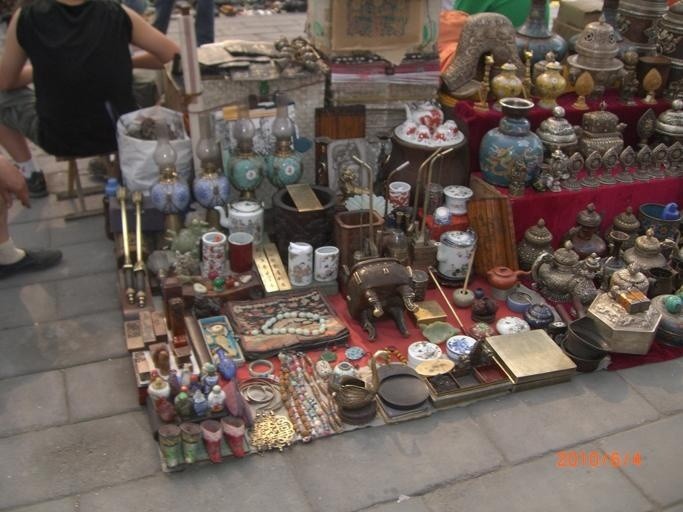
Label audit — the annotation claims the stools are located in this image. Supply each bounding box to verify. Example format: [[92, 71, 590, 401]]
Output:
[[54, 152, 118, 222]]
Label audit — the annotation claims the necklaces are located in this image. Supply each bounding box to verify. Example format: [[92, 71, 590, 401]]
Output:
[[275, 349, 342, 445], [239, 309, 326, 337]]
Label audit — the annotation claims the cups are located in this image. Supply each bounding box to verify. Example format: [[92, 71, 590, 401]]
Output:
[[289, 243, 341, 288], [202, 232, 254, 277], [390, 180, 411, 208], [444, 185, 474, 215]]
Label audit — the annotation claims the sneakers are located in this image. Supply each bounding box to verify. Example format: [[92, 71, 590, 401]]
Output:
[[23, 169, 48, 197], [88, 156, 111, 179], [0, 247, 61, 276]]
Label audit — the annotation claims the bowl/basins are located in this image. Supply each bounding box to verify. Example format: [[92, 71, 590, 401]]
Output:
[[445, 334, 478, 360]]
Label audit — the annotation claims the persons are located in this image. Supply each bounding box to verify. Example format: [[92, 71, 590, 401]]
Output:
[[0, 153, 65, 279], [151, 1, 215, 46], [0, 1, 180, 203]]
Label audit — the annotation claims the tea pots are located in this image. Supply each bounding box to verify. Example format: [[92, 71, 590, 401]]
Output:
[[530, 239, 583, 305], [487, 266, 518, 289], [434, 229, 477, 288], [566, 254, 615, 304], [624, 228, 680, 274], [213, 199, 265, 247]]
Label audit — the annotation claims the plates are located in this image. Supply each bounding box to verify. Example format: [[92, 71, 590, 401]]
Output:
[[376, 362, 430, 410]]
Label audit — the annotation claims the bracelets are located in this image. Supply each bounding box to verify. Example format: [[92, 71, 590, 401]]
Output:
[[383, 346, 409, 368]]
